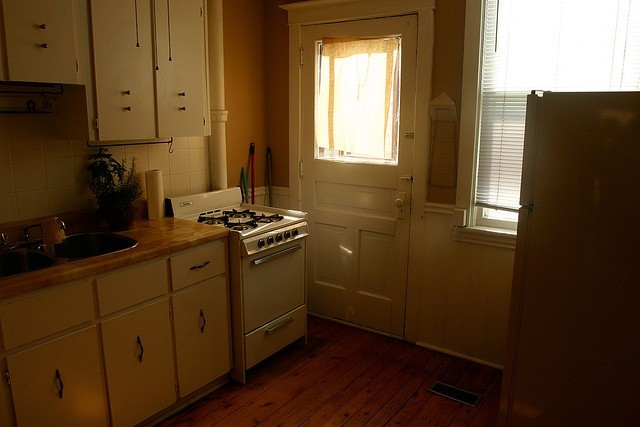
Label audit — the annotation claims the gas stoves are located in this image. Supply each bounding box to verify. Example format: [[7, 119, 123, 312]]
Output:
[[176, 203, 309, 256]]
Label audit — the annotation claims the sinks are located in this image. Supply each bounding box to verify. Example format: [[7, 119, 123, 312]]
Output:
[[15, 232, 140, 261], [0, 248, 59, 279]]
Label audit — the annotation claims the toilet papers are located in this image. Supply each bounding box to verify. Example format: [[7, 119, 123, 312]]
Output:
[[145, 169, 164, 219]]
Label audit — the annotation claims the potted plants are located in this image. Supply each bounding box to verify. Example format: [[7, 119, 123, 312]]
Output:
[[86, 146, 145, 231]]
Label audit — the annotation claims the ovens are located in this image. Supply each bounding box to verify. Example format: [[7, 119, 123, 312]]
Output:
[[229, 238, 310, 384]]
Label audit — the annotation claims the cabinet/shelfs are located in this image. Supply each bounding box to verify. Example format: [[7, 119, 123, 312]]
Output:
[[0, 251, 178, 427], [168, 240, 236, 404], [54, 0, 212, 143], [0, 0, 87, 86]]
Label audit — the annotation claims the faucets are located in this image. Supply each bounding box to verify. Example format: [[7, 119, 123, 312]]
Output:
[[21, 218, 68, 240]]
